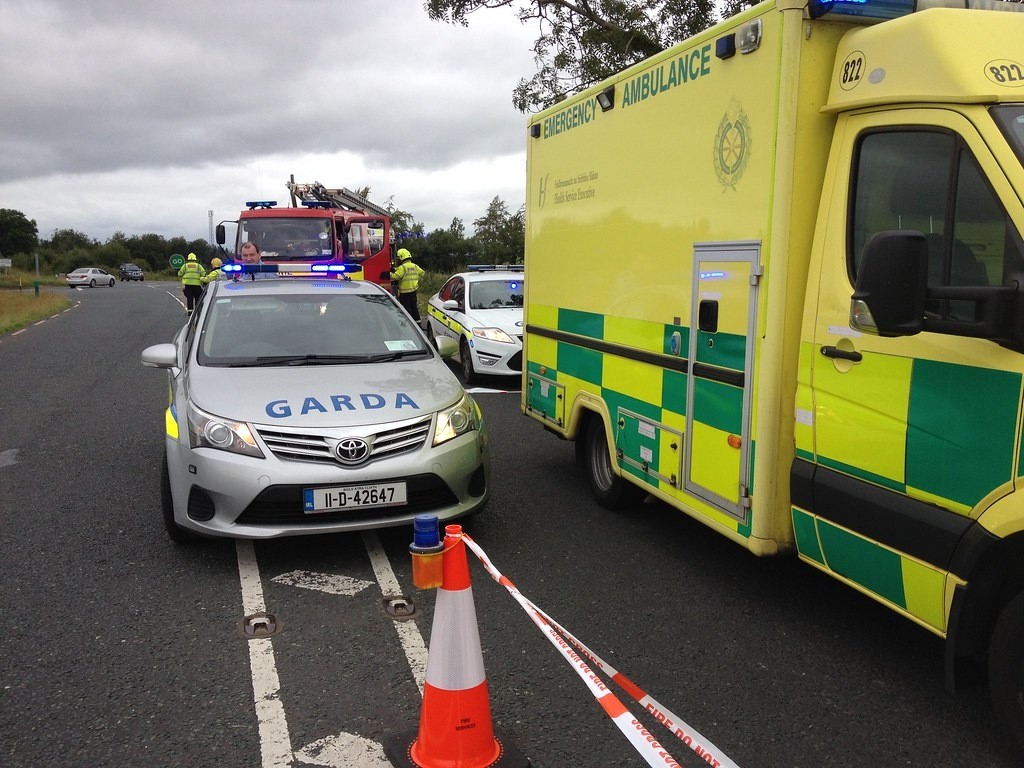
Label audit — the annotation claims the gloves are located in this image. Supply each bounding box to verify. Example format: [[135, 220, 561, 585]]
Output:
[[382, 271, 390, 279]]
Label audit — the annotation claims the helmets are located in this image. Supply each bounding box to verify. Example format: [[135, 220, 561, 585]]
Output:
[[396, 248, 407, 257], [187, 253, 197, 261], [400, 250, 412, 261], [210, 257, 222, 269]]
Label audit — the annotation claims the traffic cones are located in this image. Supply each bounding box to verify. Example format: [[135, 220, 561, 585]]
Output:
[[380, 523, 532, 768]]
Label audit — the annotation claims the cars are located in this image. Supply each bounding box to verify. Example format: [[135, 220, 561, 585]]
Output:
[[138, 262, 494, 546], [66, 267, 116, 289], [426, 264, 524, 385]]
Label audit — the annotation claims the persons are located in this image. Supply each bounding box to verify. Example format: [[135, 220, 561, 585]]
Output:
[[390, 248, 425, 330], [201, 258, 229, 282], [238, 241, 281, 280], [175, 252, 206, 316]]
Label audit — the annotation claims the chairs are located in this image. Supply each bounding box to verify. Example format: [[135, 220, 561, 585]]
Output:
[[925, 235, 994, 323]]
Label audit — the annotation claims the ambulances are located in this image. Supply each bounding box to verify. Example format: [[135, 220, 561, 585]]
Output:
[[519, 0, 1024, 760]]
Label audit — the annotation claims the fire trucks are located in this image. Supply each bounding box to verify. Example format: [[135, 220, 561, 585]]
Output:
[[214, 174, 398, 300]]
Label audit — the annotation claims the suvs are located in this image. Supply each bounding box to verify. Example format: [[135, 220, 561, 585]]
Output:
[[118, 264, 144, 281]]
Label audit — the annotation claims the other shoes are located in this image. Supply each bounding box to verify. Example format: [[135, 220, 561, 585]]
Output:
[[188, 311, 192, 315]]
